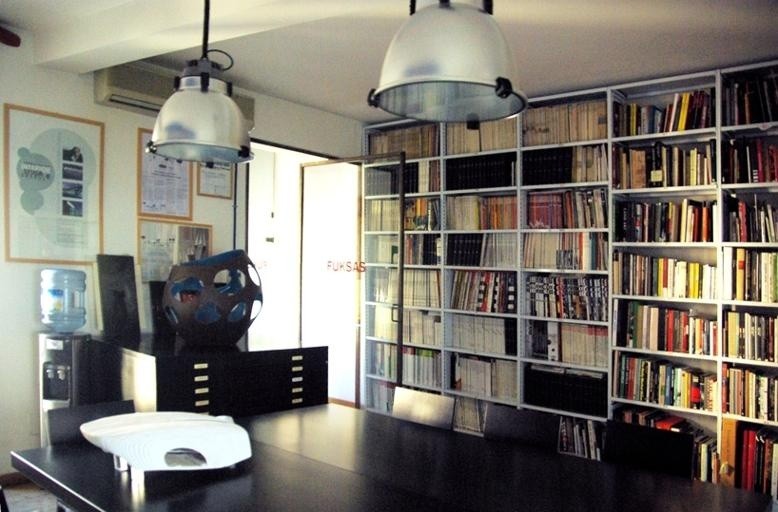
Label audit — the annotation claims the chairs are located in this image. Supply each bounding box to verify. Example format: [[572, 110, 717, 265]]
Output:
[[390, 386, 456, 430], [47, 400, 137, 445], [601, 419, 695, 482], [482, 402, 563, 454]]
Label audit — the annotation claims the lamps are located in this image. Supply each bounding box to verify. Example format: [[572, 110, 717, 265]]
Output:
[[143, 0, 253, 163], [366, 1, 528, 130]]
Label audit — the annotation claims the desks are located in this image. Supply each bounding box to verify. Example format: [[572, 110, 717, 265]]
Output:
[[85, 331, 240, 414], [10, 402, 772, 512]]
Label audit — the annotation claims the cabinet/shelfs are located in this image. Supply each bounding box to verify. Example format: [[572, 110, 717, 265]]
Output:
[[719, 61, 778, 512], [444, 102, 522, 439], [608, 70, 722, 488], [359, 117, 447, 416], [521, 86, 614, 467]]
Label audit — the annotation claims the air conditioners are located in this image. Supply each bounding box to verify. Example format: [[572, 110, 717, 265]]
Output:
[[95, 58, 255, 130]]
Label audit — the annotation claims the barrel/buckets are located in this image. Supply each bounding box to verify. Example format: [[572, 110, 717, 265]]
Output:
[[39, 266, 86, 332]]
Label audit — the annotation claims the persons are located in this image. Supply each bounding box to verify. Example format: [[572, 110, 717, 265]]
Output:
[[69, 146, 81, 161], [68, 146, 80, 161]]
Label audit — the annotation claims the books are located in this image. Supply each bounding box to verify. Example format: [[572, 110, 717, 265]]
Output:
[[521, 95, 609, 461], [366, 117, 444, 423], [610, 77, 777, 495], [446, 115, 520, 439]]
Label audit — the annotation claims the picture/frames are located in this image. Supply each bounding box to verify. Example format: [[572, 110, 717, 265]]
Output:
[[136, 127, 193, 221], [136, 219, 212, 281], [197, 160, 233, 199], [4, 103, 103, 266]]
